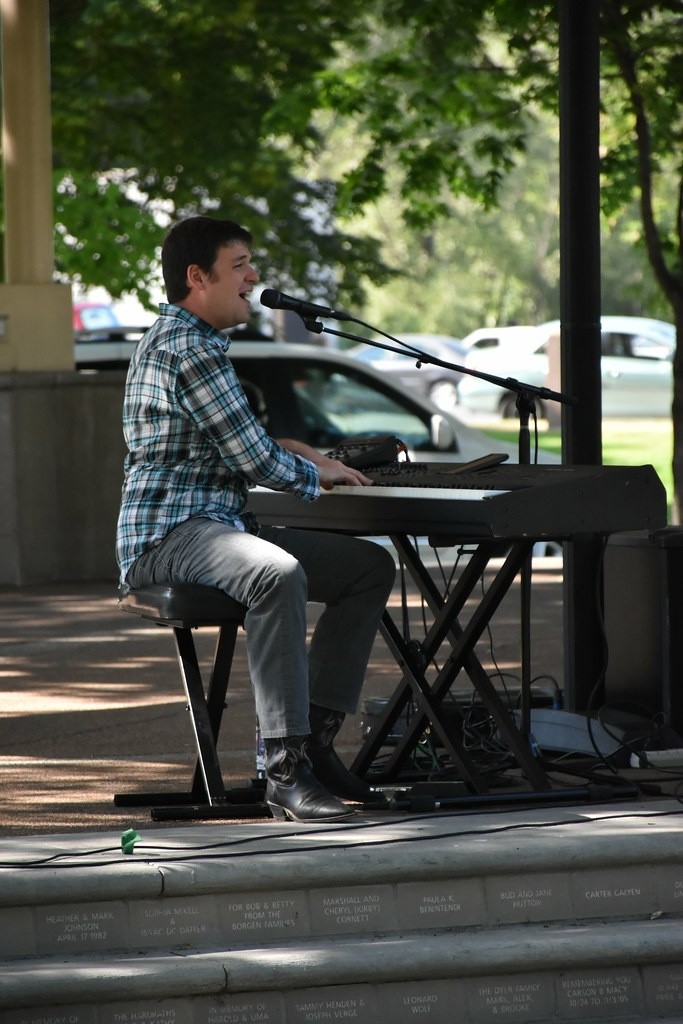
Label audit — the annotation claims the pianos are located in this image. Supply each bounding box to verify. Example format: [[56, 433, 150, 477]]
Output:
[[246, 435, 669, 815]]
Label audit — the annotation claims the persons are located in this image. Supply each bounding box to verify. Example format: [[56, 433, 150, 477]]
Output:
[[113, 217, 394, 823]]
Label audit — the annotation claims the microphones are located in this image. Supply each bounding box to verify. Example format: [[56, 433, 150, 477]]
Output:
[[260, 290, 350, 322]]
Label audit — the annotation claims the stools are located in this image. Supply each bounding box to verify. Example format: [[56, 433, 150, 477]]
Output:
[[114, 586, 274, 822]]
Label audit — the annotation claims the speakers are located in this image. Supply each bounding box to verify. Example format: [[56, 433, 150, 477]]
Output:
[[562, 524, 683, 753]]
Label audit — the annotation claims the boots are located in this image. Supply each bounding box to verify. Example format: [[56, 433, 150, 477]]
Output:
[[264, 734, 355, 824], [309, 706, 393, 806]]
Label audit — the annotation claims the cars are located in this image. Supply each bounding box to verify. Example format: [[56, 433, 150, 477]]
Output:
[[456, 315, 677, 424], [325, 333, 468, 419], [72, 325, 562, 556]]
